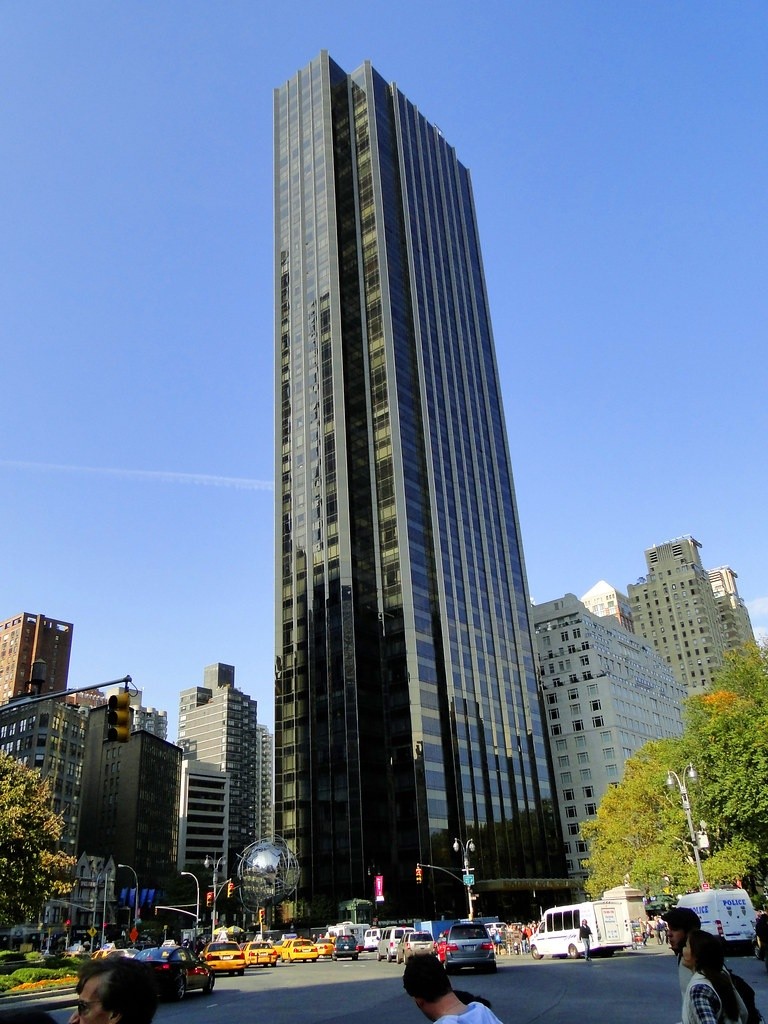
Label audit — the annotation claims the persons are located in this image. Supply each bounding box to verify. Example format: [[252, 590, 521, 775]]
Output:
[[68, 955, 160, 1024], [661, 907, 749, 1024], [311, 933, 323, 944], [755, 913, 768, 972], [681, 930, 743, 1024], [402, 953, 503, 1024], [638, 916, 669, 946], [579, 919, 593, 961], [492, 920, 541, 954]]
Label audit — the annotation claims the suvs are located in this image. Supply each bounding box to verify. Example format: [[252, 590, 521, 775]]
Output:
[[334, 934, 360, 961]]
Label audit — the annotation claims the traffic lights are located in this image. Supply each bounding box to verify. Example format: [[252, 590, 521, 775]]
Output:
[[258, 909, 266, 925], [227, 883, 234, 898], [415, 868, 424, 885], [206, 893, 213, 908], [155, 909, 158, 916], [107, 693, 131, 744]]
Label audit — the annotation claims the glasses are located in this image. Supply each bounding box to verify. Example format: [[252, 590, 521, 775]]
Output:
[[76, 1001, 101, 1016]]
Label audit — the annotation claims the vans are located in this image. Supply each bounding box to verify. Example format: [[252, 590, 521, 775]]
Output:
[[376, 926, 415, 962], [395, 930, 435, 965], [364, 929, 383, 952], [442, 922, 508, 974]]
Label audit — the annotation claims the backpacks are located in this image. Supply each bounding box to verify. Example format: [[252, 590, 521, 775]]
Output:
[[722, 963, 763, 1024]]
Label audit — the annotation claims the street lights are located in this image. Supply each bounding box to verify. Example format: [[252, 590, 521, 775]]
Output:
[[665, 761, 709, 893], [180, 872, 199, 929], [203, 855, 227, 943], [49, 898, 98, 951], [452, 837, 475, 914], [117, 864, 140, 949]]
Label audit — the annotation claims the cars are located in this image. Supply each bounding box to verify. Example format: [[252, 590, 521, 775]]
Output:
[[197, 941, 247, 977], [59, 949, 140, 959], [131, 946, 215, 1002], [239, 940, 286, 968], [282, 939, 319, 963], [314, 937, 336, 958]]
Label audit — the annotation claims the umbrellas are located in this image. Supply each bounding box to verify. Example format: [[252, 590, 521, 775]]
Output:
[[213, 925, 244, 935]]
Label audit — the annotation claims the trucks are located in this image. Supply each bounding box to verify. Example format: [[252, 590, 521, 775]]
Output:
[[675, 884, 757, 958], [528, 899, 633, 959]]
